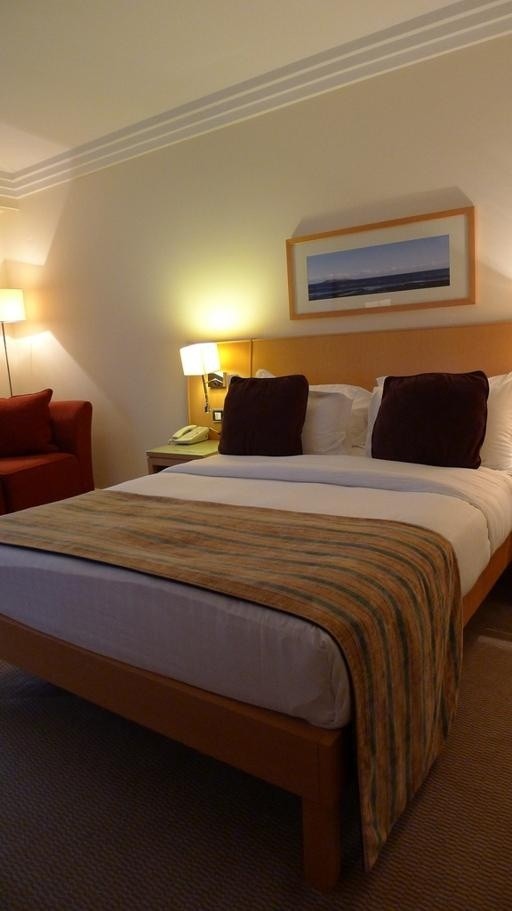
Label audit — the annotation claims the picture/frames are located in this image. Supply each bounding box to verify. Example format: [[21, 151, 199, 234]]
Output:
[[284, 204, 476, 321]]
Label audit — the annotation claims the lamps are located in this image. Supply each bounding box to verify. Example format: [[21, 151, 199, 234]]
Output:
[[178, 342, 223, 412], [0, 288, 26, 397]]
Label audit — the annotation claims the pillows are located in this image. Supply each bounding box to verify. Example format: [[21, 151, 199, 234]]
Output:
[[0, 389, 55, 457], [215, 368, 512, 473]]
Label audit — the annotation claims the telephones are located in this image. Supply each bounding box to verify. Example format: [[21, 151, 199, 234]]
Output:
[[167, 424, 209, 445]]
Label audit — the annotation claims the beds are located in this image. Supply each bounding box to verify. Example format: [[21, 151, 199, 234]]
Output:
[[0, 321, 512, 899]]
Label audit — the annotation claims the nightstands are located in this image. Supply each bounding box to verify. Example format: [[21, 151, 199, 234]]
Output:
[[145, 440, 221, 476]]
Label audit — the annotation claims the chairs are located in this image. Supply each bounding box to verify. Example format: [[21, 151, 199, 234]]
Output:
[[0, 388, 93, 519]]
